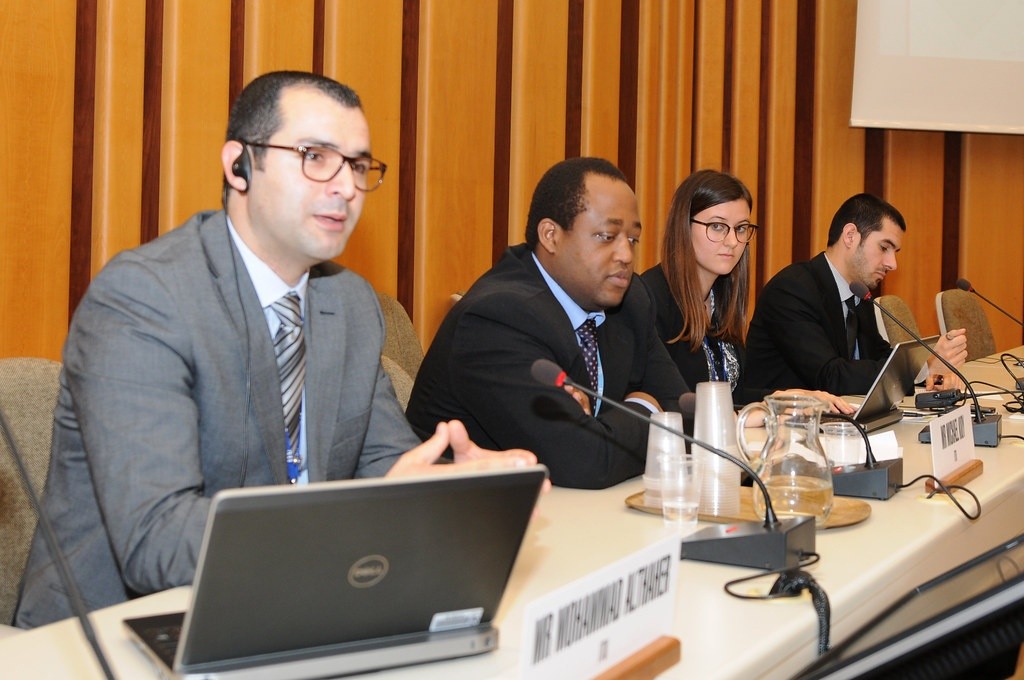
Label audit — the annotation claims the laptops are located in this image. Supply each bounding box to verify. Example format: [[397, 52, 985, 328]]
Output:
[[123, 464, 546, 680], [784, 335, 940, 435]]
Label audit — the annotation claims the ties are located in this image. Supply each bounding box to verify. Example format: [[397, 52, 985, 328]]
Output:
[[577, 319, 599, 417], [845, 294, 858, 362], [270, 290, 307, 484]]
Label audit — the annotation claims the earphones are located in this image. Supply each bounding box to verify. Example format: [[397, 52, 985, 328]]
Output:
[[233, 149, 253, 186]]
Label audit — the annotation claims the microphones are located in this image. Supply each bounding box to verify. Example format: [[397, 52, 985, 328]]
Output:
[[679, 392, 903, 500], [533, 360, 816, 570], [956, 278, 1024, 390], [849, 280, 1003, 447]]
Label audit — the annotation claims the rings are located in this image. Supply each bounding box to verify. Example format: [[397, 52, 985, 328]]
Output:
[[945, 333, 951, 341]]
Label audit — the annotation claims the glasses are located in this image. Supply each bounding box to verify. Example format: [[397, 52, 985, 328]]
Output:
[[690, 218, 760, 244], [237, 139, 386, 192]]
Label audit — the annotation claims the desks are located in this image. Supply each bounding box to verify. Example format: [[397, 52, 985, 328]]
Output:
[[0, 346, 1024, 680]]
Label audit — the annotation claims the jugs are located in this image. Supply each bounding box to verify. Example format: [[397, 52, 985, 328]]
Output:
[[736, 394, 834, 526]]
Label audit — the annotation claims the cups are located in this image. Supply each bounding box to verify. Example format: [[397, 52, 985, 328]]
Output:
[[691, 382, 741, 515], [656, 454, 700, 529], [642, 411, 686, 510], [818, 423, 865, 467]]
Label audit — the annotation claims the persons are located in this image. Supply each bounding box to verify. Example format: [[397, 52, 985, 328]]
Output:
[[745, 192, 968, 394], [639, 170, 854, 427], [402, 157, 738, 491], [11, 69, 553, 631]]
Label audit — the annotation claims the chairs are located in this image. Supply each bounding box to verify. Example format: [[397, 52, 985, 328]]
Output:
[[376, 293, 425, 413], [0, 357, 65, 626], [874, 294, 921, 349], [935, 288, 997, 362]]
